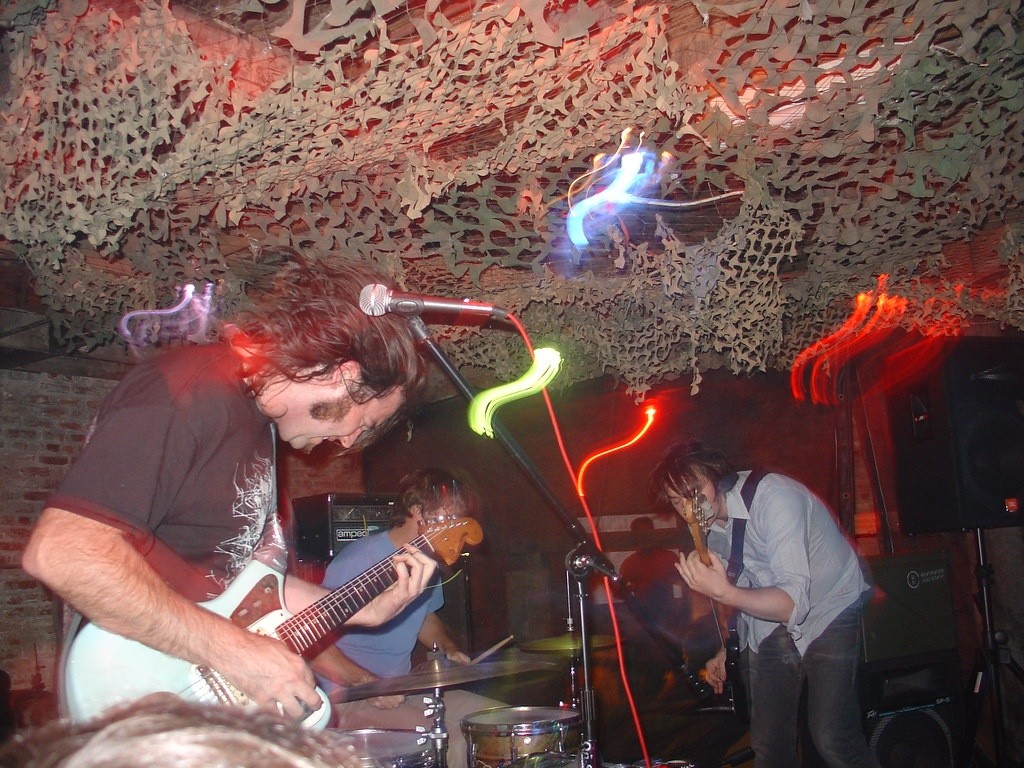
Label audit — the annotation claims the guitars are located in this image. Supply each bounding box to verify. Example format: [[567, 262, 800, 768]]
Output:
[[682, 487, 753, 726], [56, 513, 485, 733]]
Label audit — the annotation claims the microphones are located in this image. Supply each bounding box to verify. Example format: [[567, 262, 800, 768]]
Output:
[[359, 284, 508, 322]]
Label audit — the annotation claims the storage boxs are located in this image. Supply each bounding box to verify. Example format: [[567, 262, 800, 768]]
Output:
[[859, 550, 957, 665]]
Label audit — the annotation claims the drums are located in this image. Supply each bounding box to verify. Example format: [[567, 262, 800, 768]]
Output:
[[459, 705, 584, 768], [338, 728, 438, 768]]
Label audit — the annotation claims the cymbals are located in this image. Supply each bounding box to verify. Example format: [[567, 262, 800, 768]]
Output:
[[325, 658, 558, 705], [517, 631, 635, 652]]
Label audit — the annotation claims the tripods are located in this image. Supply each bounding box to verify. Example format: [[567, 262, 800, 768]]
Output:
[[966, 527, 1024, 768]]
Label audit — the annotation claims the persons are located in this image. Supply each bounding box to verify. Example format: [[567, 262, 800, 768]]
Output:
[[620, 517, 690, 640], [22, 248, 427, 719], [651, 438, 880, 768], [312, 460, 481, 710]]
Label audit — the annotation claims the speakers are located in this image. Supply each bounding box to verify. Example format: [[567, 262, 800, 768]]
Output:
[[855, 549, 965, 768], [297, 558, 475, 670], [886, 336, 1024, 536]]
